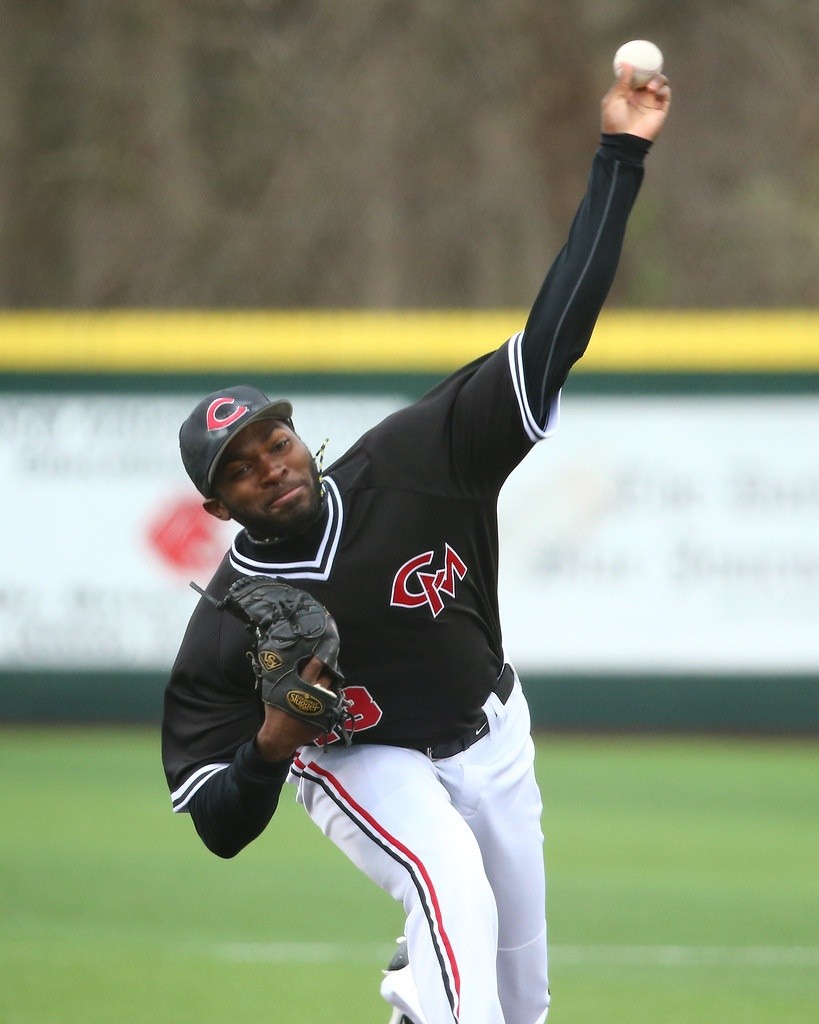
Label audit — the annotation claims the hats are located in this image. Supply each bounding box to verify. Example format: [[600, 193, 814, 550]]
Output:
[[179, 385, 293, 499]]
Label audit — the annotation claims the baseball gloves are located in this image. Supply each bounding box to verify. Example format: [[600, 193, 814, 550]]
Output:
[[223, 574, 349, 734]]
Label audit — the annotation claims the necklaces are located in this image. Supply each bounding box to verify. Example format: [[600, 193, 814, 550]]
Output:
[[262, 434, 330, 544]]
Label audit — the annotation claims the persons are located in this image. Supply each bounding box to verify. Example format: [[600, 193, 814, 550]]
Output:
[[162, 63, 672, 1024]]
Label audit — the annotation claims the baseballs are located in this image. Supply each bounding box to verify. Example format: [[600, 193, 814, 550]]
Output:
[[612, 39, 664, 88]]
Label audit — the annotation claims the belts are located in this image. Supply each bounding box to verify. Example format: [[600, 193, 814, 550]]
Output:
[[426, 663, 514, 760]]
